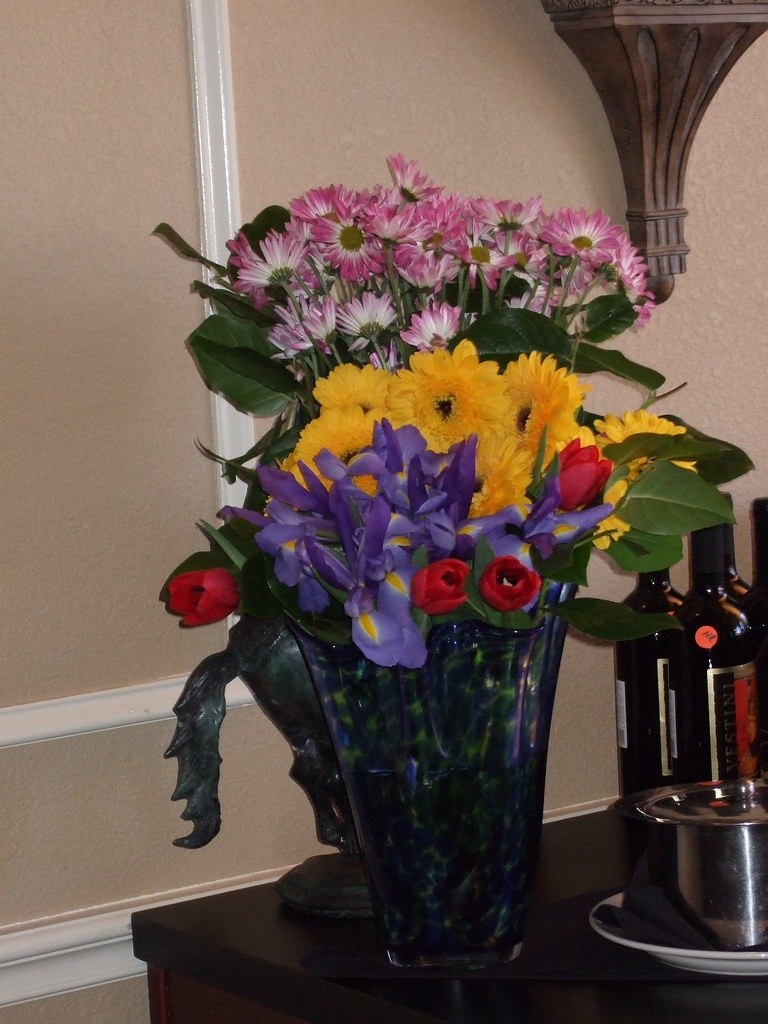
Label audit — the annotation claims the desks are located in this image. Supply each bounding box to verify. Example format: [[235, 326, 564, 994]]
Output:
[[130, 813, 768, 1024]]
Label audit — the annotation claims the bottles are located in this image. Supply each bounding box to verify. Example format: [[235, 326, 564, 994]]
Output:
[[615, 493, 768, 799]]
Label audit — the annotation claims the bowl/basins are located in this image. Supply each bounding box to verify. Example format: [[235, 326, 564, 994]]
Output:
[[613, 779, 768, 950]]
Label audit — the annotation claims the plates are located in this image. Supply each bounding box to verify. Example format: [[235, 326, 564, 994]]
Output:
[[589, 893, 768, 976]]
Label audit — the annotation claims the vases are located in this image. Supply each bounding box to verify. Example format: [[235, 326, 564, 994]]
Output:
[[164, 611, 365, 928], [275, 552, 589, 1003]]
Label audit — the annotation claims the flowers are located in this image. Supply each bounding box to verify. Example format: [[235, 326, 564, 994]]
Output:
[[229, 335, 687, 670], [148, 152, 755, 650]]
